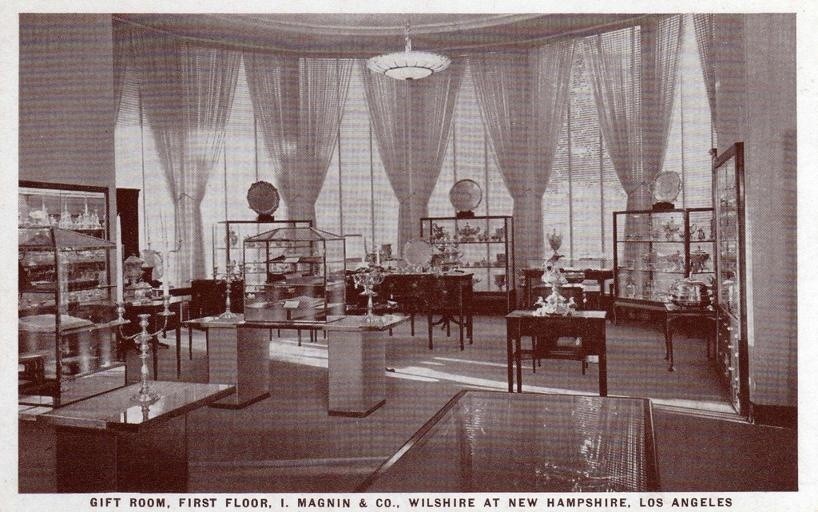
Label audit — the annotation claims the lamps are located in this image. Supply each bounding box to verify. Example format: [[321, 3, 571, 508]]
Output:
[[365, 17, 453, 83]]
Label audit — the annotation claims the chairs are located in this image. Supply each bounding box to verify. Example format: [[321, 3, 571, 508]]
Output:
[[347, 267, 475, 352]]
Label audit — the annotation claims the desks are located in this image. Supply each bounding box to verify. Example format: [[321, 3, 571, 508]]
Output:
[[351, 389, 660, 492], [19, 377, 236, 491], [182, 312, 412, 418], [505, 254, 609, 396]]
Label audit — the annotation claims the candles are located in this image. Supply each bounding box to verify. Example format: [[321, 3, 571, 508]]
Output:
[[111, 213, 130, 323], [156, 233, 175, 316]]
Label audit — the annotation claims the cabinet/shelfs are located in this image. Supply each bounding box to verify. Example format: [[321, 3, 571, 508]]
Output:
[[612, 140, 747, 416], [18, 180, 126, 409], [420, 216, 516, 324], [212, 218, 348, 324]]
